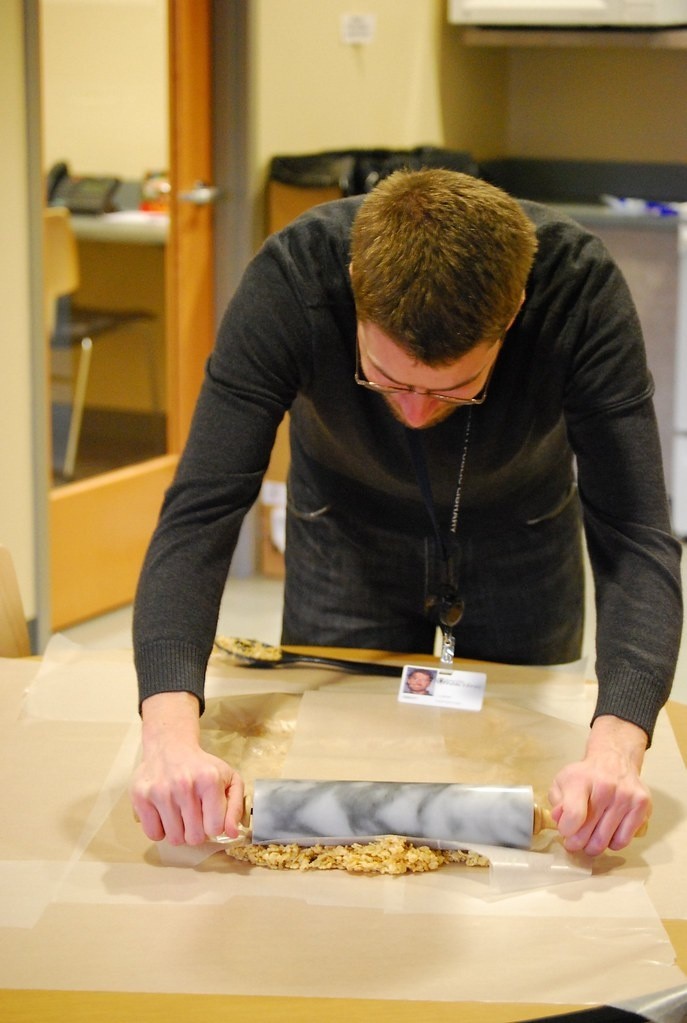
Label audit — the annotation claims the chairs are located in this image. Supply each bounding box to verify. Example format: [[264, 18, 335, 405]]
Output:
[[46, 205, 154, 485]]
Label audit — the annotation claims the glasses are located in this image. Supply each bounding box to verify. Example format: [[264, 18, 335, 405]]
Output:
[[354, 340, 503, 406]]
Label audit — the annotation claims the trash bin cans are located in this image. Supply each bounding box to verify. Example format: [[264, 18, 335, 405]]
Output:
[[229, 503, 257, 580]]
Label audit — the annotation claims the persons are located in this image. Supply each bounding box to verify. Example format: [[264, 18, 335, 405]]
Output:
[[127, 166, 684, 850], [405, 669, 437, 695]]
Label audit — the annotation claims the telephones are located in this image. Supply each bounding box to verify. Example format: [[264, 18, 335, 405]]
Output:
[[43, 162, 120, 215]]
